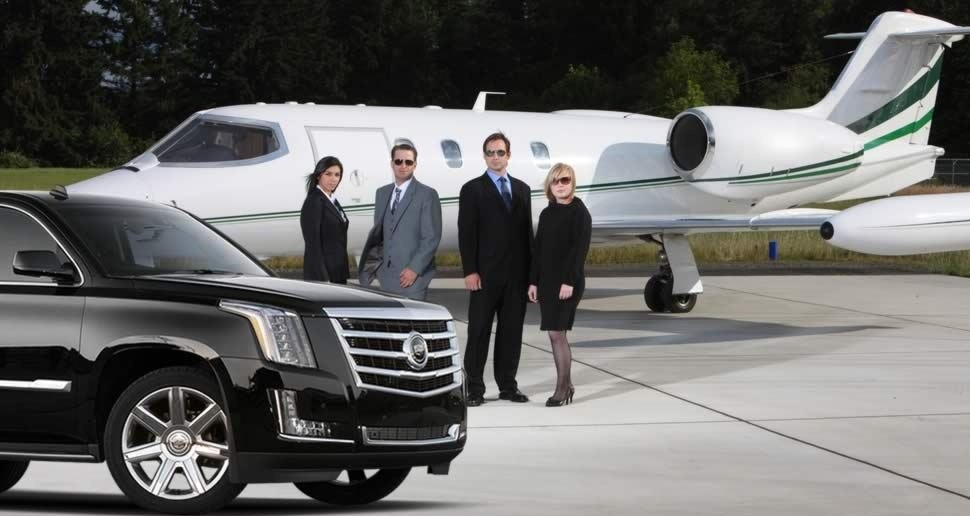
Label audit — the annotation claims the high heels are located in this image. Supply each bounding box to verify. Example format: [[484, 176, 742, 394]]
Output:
[[549, 385, 574, 403], [546, 387, 571, 406]]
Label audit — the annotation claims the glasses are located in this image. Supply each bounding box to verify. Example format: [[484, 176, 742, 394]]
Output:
[[550, 177, 572, 185], [485, 150, 508, 157], [395, 159, 413, 166]]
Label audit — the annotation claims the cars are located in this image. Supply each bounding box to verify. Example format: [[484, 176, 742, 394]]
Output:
[[0, 188, 473, 516]]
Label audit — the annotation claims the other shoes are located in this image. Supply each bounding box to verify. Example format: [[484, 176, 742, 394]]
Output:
[[500, 387, 526, 402], [466, 395, 484, 406]]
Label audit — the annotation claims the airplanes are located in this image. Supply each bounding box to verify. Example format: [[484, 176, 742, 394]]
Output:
[[63, 9, 969, 313]]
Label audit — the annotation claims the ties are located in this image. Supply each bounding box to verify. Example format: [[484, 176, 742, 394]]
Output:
[[391, 188, 402, 215], [499, 177, 511, 213]]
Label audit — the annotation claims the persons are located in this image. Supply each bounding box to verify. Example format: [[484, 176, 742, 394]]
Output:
[[358, 144, 443, 302], [527, 162, 592, 407], [300, 155, 351, 284], [456, 132, 536, 406]]
